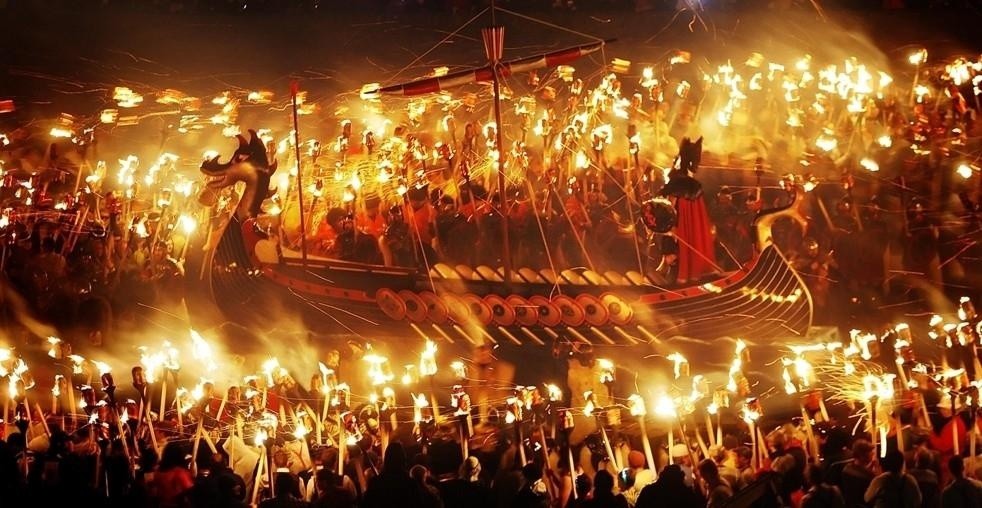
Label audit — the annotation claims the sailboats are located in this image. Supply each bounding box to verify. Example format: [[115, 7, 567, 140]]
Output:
[[198, 2, 817, 353]]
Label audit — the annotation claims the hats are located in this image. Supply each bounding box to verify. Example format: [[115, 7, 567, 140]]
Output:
[[732, 446, 751, 457], [629, 450, 645, 467], [465, 455, 479, 467]]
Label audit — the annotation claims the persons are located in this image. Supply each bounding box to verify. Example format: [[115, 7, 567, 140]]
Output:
[[0, 95, 982, 508]]
